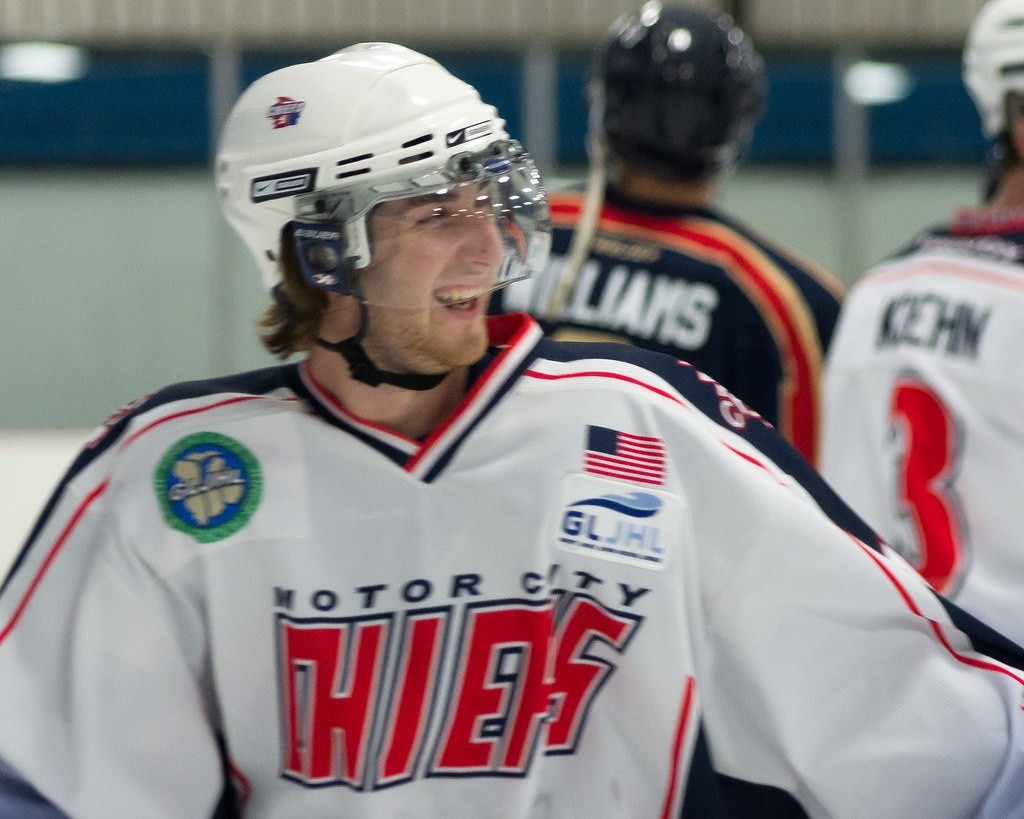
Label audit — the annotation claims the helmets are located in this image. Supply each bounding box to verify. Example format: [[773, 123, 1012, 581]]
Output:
[[964, 1, 1024, 134], [592, 1, 763, 178], [218, 41, 552, 312]]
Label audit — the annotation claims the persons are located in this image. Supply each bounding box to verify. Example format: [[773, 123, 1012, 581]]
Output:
[[482, 1, 849, 469], [814, 0, 1024, 646], [1, 42, 1024, 818]]
